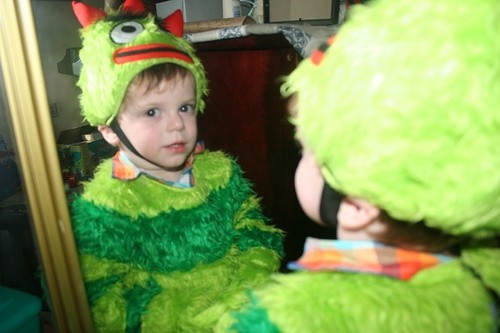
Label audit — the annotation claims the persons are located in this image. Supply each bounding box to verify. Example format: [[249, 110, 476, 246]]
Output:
[[185, 2, 500, 333], [62, 0, 284, 333]]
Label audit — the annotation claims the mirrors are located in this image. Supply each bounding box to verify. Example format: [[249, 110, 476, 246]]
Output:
[[0, 0, 354, 333]]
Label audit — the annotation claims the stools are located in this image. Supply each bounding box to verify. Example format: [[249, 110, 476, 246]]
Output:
[[0, 283, 44, 333]]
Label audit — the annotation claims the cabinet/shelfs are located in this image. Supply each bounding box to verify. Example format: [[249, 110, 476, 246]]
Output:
[[181, 23, 336, 276]]
